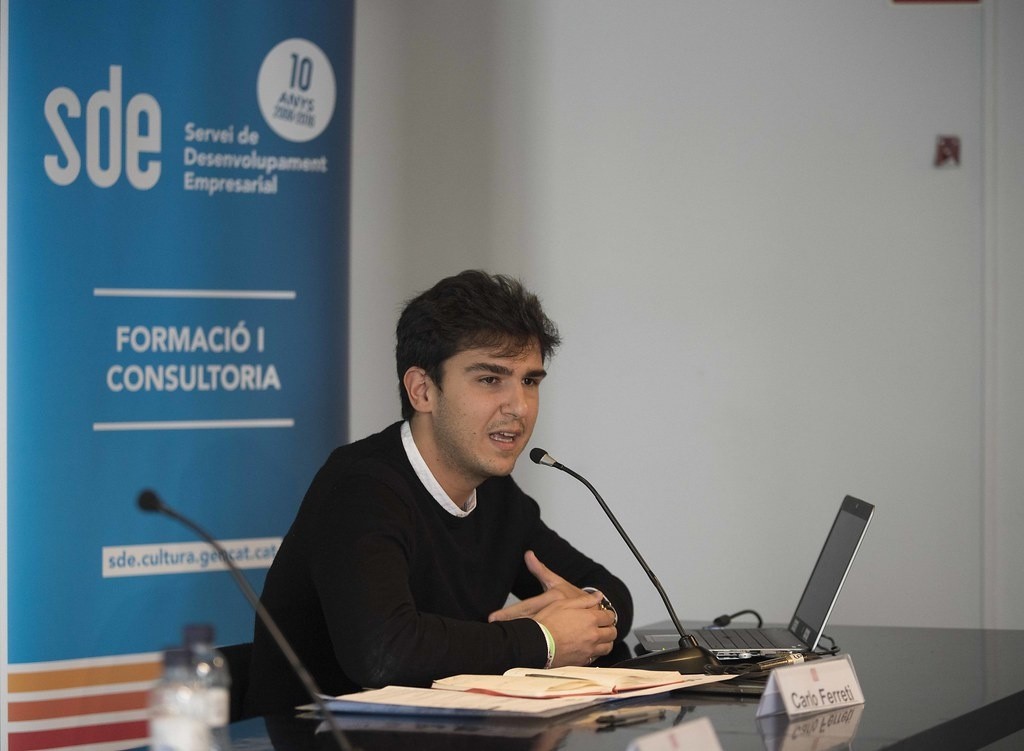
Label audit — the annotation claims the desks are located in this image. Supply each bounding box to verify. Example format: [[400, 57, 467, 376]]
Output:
[[122, 619, 1024, 751]]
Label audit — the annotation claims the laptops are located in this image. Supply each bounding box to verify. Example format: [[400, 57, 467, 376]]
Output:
[[634, 495, 875, 658]]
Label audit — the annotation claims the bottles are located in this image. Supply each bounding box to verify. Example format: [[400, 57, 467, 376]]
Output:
[[185, 624, 230, 751], [147, 651, 213, 751]]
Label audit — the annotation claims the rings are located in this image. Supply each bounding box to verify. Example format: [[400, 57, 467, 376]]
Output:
[[588, 657, 594, 664]]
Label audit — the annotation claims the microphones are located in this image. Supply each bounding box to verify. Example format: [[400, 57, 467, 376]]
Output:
[[529, 448, 720, 677], [138, 488, 352, 751]]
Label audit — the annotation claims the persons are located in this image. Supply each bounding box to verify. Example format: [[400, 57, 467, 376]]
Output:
[[253, 268, 634, 751]]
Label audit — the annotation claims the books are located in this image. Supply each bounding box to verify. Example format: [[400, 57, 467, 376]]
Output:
[[431, 667, 685, 701]]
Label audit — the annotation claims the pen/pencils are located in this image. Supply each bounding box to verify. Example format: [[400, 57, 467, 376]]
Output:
[[592, 708, 666, 725]]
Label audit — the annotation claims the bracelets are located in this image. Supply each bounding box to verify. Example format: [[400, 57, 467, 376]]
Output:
[[582, 589, 618, 627]]
